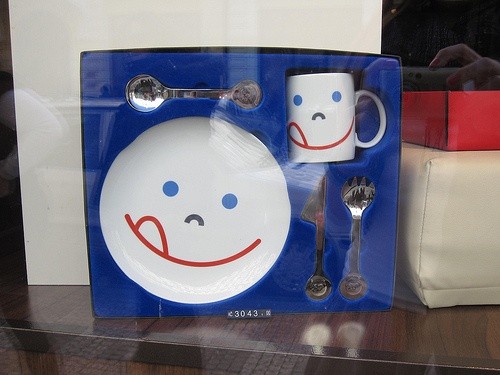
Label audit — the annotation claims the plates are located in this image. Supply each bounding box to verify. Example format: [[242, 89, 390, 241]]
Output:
[[98, 116, 292, 305]]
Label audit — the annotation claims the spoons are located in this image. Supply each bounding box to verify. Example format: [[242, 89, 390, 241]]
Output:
[[121, 71, 264, 114]]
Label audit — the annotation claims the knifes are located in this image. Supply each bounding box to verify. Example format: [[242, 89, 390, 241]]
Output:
[[299, 173, 331, 303]]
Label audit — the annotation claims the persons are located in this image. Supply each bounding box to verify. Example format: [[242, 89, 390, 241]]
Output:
[[349, 43, 500, 149]]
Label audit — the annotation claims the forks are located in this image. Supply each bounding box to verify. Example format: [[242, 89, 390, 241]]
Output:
[[337, 177, 375, 303]]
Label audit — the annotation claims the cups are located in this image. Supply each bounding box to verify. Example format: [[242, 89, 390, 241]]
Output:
[[287, 70, 387, 165]]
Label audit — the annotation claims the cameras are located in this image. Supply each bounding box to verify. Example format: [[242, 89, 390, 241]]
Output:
[[380, 65, 477, 120]]
